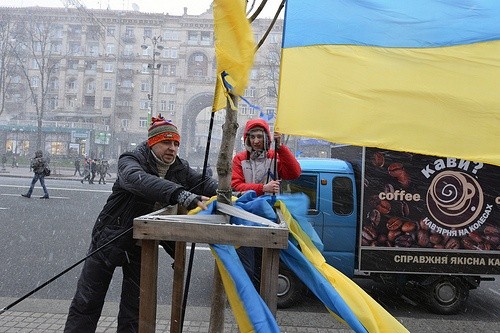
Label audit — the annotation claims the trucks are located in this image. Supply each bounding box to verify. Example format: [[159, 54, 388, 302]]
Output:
[[275, 145, 500, 316]]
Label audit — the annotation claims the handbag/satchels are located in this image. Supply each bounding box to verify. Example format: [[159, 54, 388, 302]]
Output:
[[44, 168, 50, 176]]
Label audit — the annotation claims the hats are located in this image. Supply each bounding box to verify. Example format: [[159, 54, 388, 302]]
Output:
[[148, 113, 180, 148], [244, 119, 272, 147]]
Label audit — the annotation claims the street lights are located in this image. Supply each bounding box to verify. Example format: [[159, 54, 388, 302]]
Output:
[[140, 33, 164, 123]]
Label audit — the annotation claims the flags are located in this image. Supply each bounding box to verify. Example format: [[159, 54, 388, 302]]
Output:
[[274, 0, 500, 167], [211, 0, 253, 113]]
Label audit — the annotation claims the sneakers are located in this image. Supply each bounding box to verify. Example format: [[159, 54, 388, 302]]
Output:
[[21, 194, 30, 198], [40, 196, 49, 199]]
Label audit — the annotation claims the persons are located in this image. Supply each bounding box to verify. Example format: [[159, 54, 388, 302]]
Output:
[[63, 113, 218, 333], [230, 119, 301, 295], [81, 158, 111, 184], [21, 149, 49, 199], [206, 163, 213, 178], [74, 157, 81, 176], [45, 153, 57, 174], [2, 153, 7, 171], [12, 155, 19, 167]]
